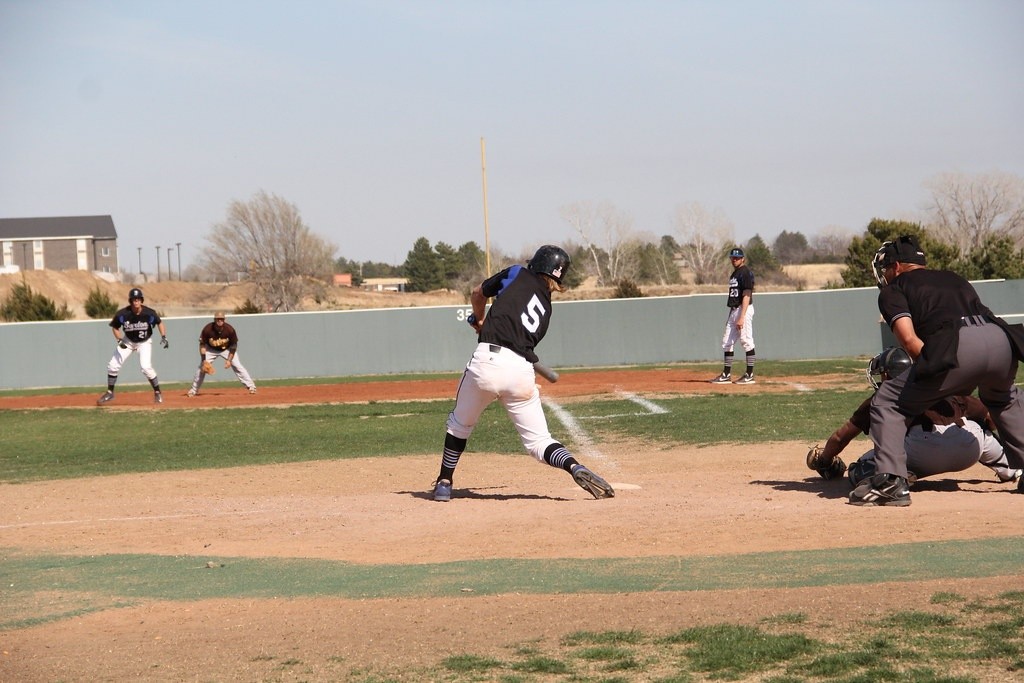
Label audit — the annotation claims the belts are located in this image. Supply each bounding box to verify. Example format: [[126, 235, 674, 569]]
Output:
[[489, 344, 500, 352], [960, 314, 995, 326], [921, 418, 965, 433], [731, 306, 738, 310]]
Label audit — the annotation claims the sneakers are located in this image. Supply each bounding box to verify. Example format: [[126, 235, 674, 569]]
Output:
[[710, 372, 732, 384], [571, 465, 615, 499], [855, 461, 917, 488], [848, 473, 912, 506], [998, 468, 1022, 483], [734, 372, 756, 384], [432, 481, 451, 501]]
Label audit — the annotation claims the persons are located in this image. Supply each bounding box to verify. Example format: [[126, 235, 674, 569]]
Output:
[[185, 312, 257, 397], [847, 235, 1024, 506], [710, 248, 756, 384], [806, 349, 1022, 488], [99, 288, 169, 404], [431, 245, 615, 501]]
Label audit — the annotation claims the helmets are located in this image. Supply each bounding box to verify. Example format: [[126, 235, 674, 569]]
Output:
[[214, 311, 226, 319], [865, 348, 914, 391], [528, 245, 570, 285], [729, 249, 745, 257], [129, 289, 144, 303]]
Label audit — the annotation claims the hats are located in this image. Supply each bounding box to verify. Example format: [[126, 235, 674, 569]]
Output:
[[882, 240, 926, 268]]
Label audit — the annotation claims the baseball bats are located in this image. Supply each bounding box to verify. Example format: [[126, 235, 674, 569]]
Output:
[[467, 315, 560, 383]]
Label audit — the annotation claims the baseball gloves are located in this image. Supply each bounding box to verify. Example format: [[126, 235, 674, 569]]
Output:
[[200, 361, 215, 375], [806, 446, 847, 479]]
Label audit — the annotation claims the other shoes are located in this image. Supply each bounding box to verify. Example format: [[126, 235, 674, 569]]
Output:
[[249, 387, 257, 393], [101, 391, 114, 402], [155, 392, 162, 404], [188, 388, 198, 397]]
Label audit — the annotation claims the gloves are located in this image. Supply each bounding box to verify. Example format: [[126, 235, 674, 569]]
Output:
[[118, 338, 129, 349], [160, 336, 169, 350]]
